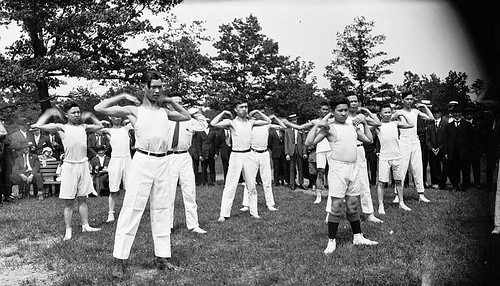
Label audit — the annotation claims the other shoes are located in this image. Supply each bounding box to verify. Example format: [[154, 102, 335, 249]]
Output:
[[62, 230, 70, 241], [112, 258, 123, 278], [81, 226, 102, 232], [324, 239, 336, 254], [106, 214, 114, 222], [491, 227, 500, 233], [353, 239, 379, 246], [217, 176, 480, 223], [156, 258, 174, 270], [191, 227, 207, 234]]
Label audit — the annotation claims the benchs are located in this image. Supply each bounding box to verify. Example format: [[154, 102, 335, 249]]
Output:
[[23, 162, 99, 198]]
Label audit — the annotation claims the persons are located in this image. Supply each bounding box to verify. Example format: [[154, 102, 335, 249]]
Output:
[[210, 99, 272, 223], [94, 69, 191, 278], [34, 103, 105, 241], [304, 97, 379, 253], [0, 89, 500, 233], [89, 115, 134, 222]]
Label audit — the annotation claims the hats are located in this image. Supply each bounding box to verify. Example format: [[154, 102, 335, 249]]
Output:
[[287, 114, 299, 121], [100, 120, 111, 127], [415, 100, 432, 107], [15, 118, 53, 156], [448, 101, 458, 107]]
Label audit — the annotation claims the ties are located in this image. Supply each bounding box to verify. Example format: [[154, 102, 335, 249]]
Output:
[[172, 120, 180, 148], [435, 120, 438, 128], [293, 128, 295, 144], [456, 121, 460, 127], [226, 130, 230, 144], [96, 134, 99, 146], [24, 153, 28, 170]]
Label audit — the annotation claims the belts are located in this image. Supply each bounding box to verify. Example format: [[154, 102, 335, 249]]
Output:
[[251, 148, 268, 153], [137, 149, 166, 157], [232, 149, 251, 152], [357, 144, 363, 147], [168, 150, 187, 154]]
